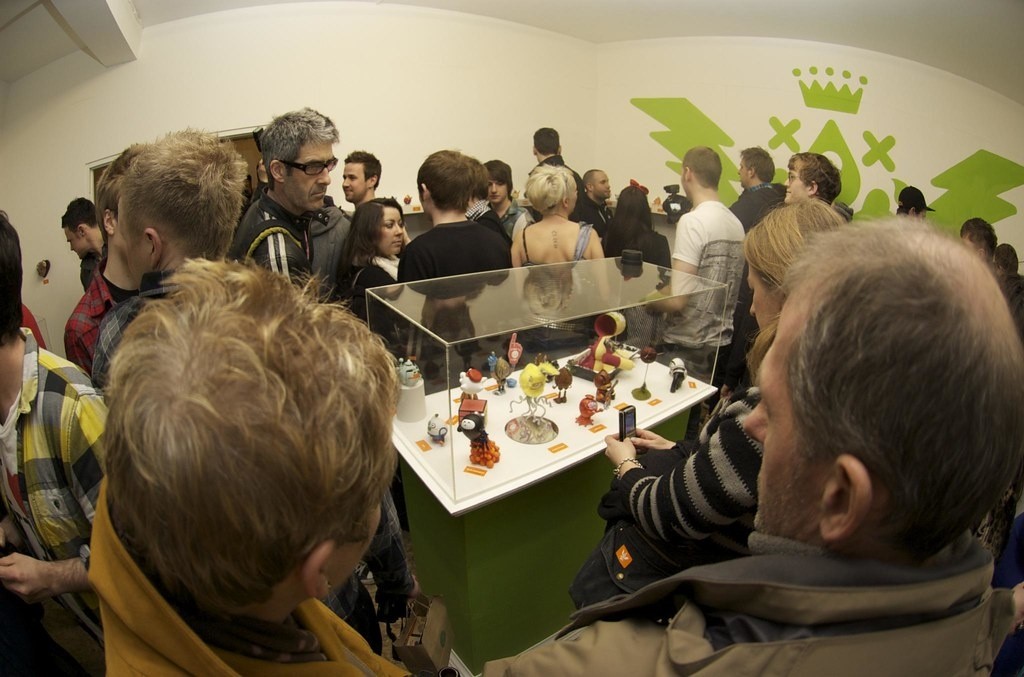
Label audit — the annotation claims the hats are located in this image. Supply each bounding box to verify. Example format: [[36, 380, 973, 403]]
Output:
[[898, 186, 936, 212]]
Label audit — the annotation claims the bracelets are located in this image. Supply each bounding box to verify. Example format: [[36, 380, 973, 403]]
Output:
[[613, 458, 644, 480]]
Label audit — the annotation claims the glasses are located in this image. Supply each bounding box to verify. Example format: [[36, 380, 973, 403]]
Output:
[[280, 155, 338, 175], [789, 172, 799, 181]]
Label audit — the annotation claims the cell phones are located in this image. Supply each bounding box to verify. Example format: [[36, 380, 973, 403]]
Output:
[[619, 405, 637, 440]]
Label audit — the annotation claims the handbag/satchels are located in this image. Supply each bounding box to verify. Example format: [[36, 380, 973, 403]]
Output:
[[569, 520, 690, 611], [392, 597, 455, 677]]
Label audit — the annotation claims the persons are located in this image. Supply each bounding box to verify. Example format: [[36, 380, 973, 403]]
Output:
[[480, 213, 1024, 677], [88, 259, 412, 677], [0, 109, 1024, 677], [641, 145, 746, 381]]
